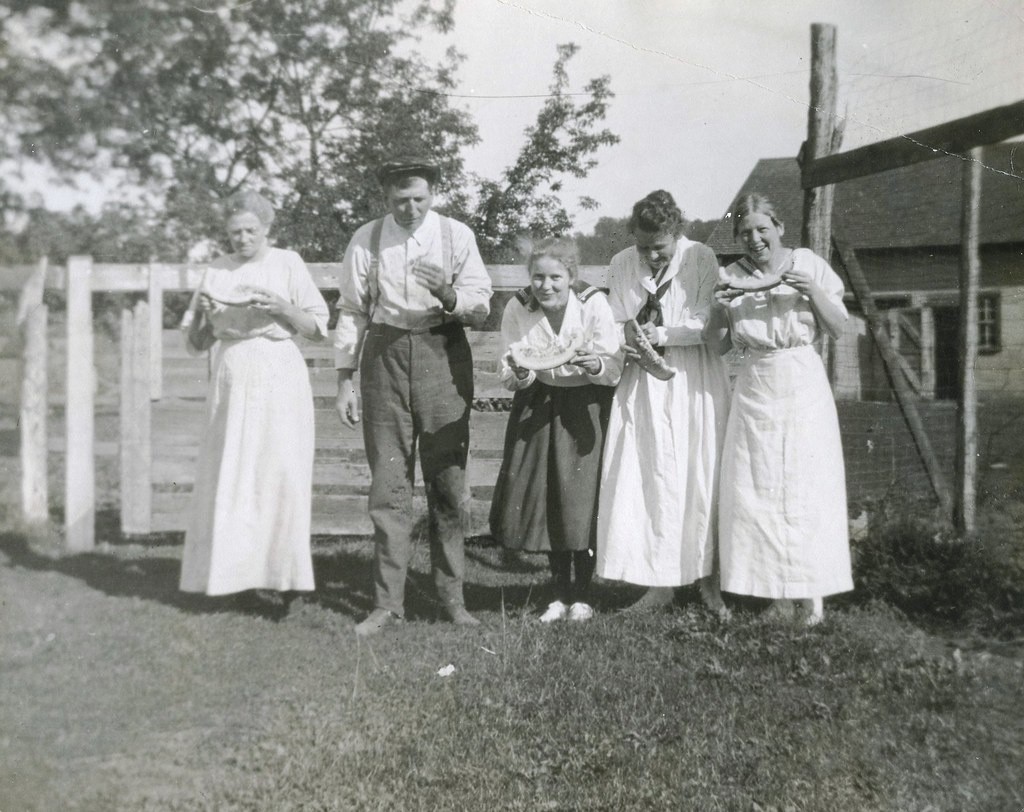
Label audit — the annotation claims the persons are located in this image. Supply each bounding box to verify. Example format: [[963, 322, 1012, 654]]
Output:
[[488, 237, 625, 623], [710, 193, 855, 633], [179, 191, 329, 620], [333, 156, 494, 636], [597, 190, 732, 621]]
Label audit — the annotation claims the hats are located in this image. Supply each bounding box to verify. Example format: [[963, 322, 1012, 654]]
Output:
[[378, 156, 438, 187]]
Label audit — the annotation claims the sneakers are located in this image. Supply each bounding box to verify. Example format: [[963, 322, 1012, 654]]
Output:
[[568, 602, 593, 624], [538, 599, 569, 624]]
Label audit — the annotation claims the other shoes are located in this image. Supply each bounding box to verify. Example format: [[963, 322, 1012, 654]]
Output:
[[606, 595, 678, 628], [434, 605, 481, 628], [352, 607, 401, 636], [699, 596, 741, 634]]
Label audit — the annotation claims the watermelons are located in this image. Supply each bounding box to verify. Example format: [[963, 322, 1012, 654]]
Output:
[[624, 318, 677, 381], [509, 338, 583, 371], [717, 257, 795, 292], [200, 284, 257, 307]]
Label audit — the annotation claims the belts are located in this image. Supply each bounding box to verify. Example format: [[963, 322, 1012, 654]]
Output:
[[383, 324, 445, 335]]
